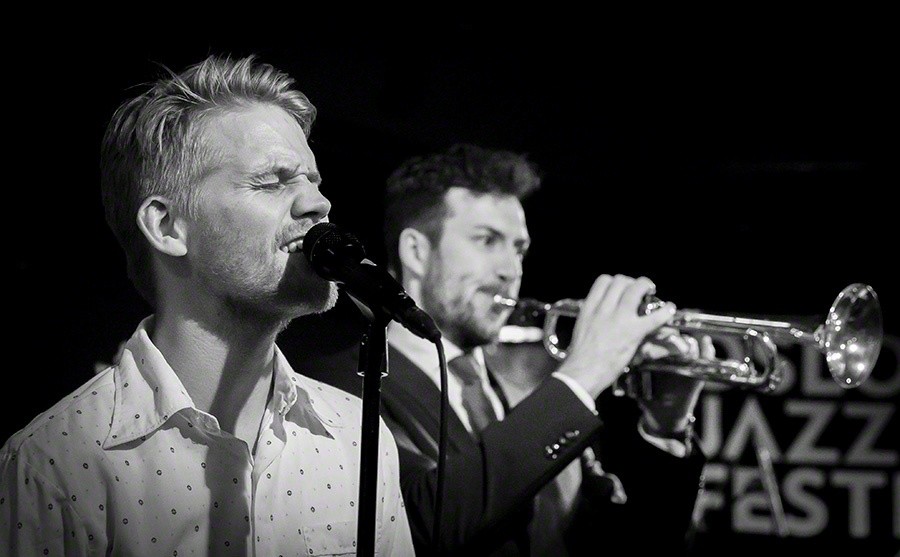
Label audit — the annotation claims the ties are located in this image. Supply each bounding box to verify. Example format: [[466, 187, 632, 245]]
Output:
[[449, 357, 498, 436]]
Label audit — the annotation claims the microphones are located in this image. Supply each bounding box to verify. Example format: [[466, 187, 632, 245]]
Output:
[[303, 222, 441, 342]]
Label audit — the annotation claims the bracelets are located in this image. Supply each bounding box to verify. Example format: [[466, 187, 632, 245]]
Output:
[[641, 419, 692, 441]]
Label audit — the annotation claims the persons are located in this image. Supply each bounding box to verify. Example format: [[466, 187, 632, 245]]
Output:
[[0, 53, 418, 557], [315, 140, 714, 557]]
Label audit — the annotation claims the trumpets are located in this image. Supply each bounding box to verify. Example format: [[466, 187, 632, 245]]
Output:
[[491, 283, 885, 397]]
